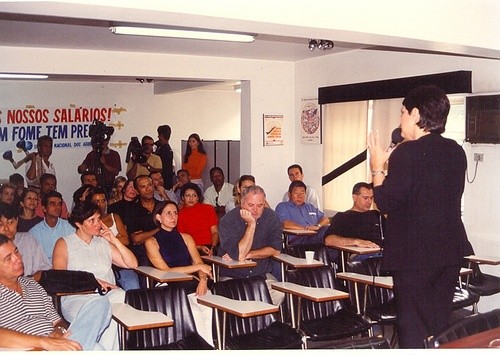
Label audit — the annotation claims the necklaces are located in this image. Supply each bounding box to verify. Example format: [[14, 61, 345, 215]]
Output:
[[85, 239, 91, 243]]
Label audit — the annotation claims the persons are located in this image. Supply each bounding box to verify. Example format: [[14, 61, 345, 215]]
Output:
[[367, 83, 474, 349], [0, 125, 386, 350]]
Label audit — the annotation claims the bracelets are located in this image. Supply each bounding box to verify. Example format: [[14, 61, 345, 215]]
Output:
[[57, 326, 67, 334], [372, 170, 385, 176], [147, 165, 151, 169], [201, 275, 208, 280], [315, 224, 321, 228]]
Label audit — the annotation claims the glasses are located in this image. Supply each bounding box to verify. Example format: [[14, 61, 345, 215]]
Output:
[[216, 197, 219, 206]]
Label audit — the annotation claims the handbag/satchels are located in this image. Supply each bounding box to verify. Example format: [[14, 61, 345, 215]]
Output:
[[39, 270, 101, 296]]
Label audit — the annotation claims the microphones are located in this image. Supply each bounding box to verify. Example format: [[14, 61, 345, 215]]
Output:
[[387, 128, 404, 152]]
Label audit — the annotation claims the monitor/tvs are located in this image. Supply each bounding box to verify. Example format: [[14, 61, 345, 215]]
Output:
[[466, 94, 500, 144]]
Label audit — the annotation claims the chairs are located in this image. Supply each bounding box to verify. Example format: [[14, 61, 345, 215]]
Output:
[[197, 276, 303, 350], [328, 239, 500, 349], [271, 266, 372, 349], [110, 285, 214, 350]]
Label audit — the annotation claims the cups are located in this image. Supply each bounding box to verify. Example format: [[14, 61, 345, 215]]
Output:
[[305, 251, 315, 264]]
